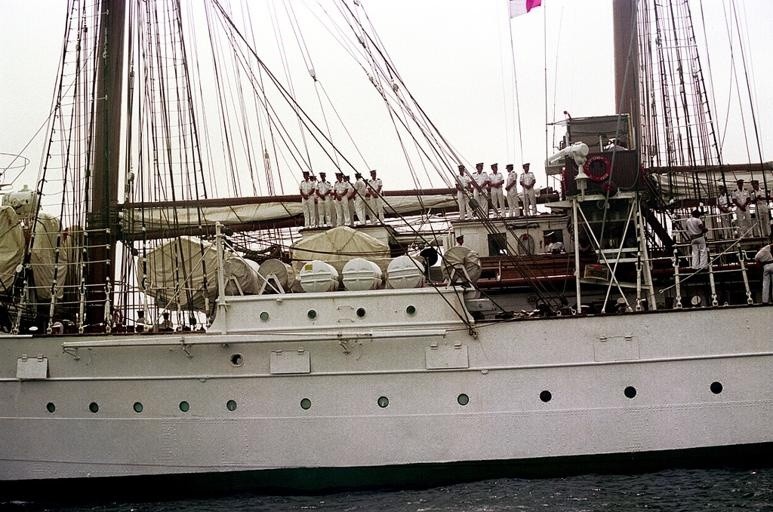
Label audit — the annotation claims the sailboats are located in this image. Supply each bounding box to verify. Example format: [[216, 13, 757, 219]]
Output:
[[1, 0, 773, 511]]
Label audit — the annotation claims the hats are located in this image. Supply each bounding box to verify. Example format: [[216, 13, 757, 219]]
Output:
[[458, 163, 530, 169], [455, 234, 464, 239], [302, 170, 376, 179], [716, 178, 759, 189]]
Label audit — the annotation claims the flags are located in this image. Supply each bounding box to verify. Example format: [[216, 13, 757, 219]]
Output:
[[510, 0, 542, 18]]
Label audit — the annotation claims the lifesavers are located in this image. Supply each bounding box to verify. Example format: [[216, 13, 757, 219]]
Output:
[[585, 156, 611, 181]]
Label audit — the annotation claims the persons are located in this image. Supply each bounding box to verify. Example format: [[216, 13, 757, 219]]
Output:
[[161, 313, 173, 328], [300, 170, 384, 229], [686, 179, 773, 304], [455, 162, 537, 220], [455, 234, 465, 247], [136, 311, 148, 323]]
